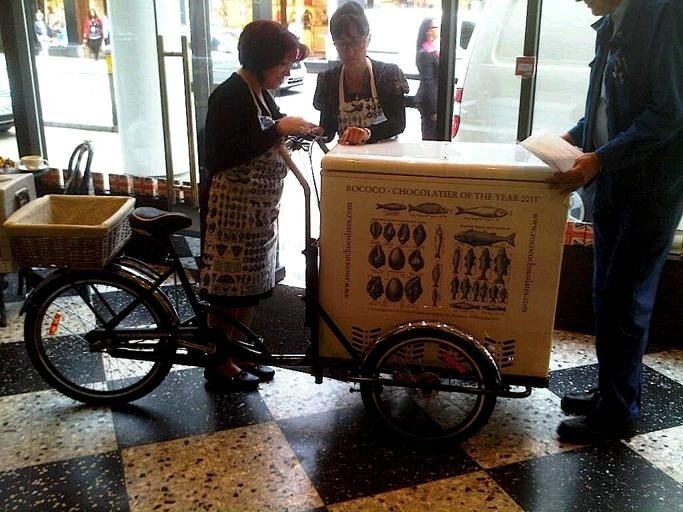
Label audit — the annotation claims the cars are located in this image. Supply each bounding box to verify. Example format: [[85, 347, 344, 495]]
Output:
[[183, 0, 454, 105]]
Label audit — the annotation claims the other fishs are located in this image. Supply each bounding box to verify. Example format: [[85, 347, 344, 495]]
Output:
[[455, 206, 508, 217], [432, 289, 439, 307], [408, 203, 447, 214], [452, 229, 516, 247], [431, 263, 440, 288], [432, 223, 443, 259], [449, 246, 511, 312], [376, 203, 407, 210]]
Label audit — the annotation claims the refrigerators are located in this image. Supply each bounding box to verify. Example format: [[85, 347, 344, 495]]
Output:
[[315, 138, 571, 381]]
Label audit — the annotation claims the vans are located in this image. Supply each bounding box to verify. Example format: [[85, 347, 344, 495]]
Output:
[[439, 0, 683, 351]]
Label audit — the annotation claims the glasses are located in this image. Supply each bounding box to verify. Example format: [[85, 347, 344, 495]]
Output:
[[334, 38, 369, 51]]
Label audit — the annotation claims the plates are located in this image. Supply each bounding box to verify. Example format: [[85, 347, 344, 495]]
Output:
[[17, 164, 48, 171]]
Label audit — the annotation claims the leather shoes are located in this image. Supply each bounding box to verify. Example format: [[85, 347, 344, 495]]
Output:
[[204, 364, 274, 388]]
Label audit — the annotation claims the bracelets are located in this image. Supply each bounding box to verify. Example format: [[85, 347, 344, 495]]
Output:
[[364, 126, 372, 139]]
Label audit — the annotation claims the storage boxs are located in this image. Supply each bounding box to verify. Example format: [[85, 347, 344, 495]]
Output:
[[1, 171, 41, 260]]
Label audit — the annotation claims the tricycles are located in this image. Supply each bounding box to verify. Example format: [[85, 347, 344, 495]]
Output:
[[15, 134, 552, 449]]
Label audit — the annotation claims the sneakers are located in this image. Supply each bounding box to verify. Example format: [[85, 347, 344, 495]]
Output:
[[562, 388, 601, 413], [558, 416, 637, 444]]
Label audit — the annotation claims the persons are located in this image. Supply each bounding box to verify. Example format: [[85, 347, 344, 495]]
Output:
[[196, 19, 313, 389], [310, 1, 409, 146], [32, 4, 109, 62], [415, 16, 440, 141], [543, 1, 681, 444]]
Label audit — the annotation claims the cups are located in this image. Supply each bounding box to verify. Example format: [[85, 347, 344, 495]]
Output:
[[19, 154, 48, 169]]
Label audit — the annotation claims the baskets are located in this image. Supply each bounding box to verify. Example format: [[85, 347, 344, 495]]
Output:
[[3, 194, 136, 269]]
